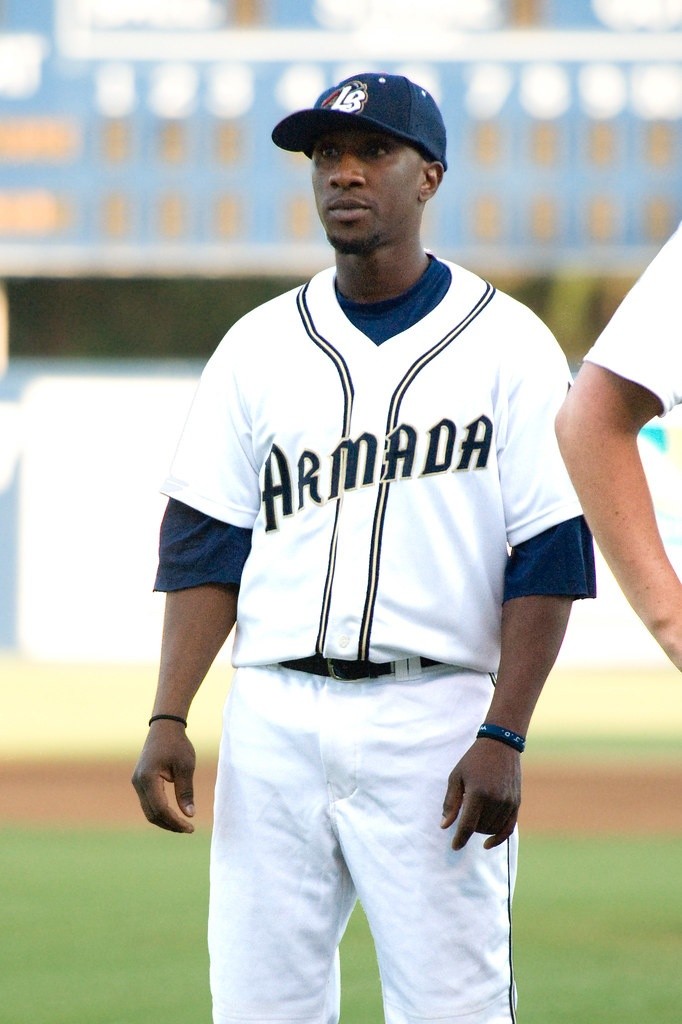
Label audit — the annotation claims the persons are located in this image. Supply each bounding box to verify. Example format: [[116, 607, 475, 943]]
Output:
[[556, 220, 682, 666], [131, 73, 597, 1022]]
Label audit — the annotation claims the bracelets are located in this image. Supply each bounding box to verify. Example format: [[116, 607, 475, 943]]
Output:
[[149, 714, 187, 729], [476, 725, 527, 753]]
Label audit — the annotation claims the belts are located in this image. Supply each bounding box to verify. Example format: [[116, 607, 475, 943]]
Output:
[[278, 653, 444, 681]]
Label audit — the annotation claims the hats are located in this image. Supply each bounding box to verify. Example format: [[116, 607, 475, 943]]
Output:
[[271, 73, 448, 172]]
